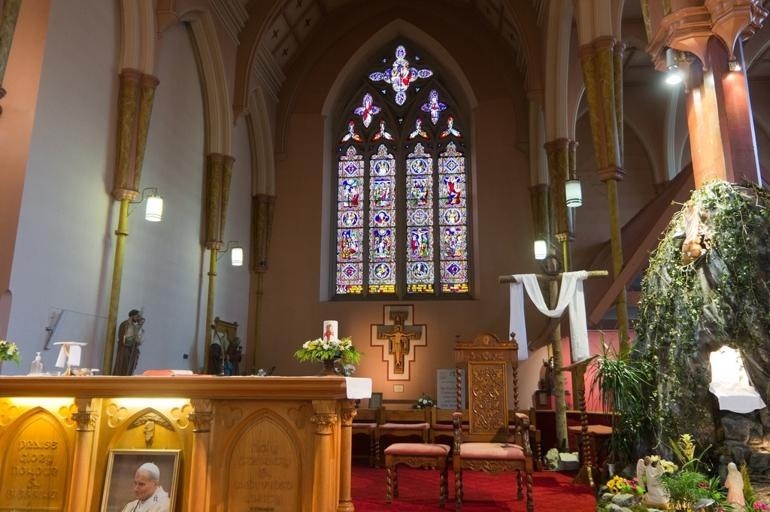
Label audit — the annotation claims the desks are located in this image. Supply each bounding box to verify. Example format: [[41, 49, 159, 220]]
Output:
[[0, 374, 355, 511]]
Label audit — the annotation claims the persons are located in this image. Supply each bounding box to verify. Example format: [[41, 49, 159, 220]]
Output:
[[224, 336, 243, 375], [383, 327, 416, 366], [643, 455, 668, 504], [128, 317, 146, 375], [724, 462, 745, 507], [122, 462, 171, 512], [114, 310, 140, 375], [223, 353, 234, 376], [208, 343, 226, 376]]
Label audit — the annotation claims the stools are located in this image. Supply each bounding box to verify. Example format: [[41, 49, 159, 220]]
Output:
[[383, 441, 452, 508]]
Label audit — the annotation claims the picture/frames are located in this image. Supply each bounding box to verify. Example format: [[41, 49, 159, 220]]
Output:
[[369, 392, 382, 411], [96, 448, 183, 511]]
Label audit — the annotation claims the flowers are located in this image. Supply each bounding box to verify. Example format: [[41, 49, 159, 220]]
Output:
[[607, 473, 649, 496], [292, 336, 362, 368], [0, 338, 23, 369], [654, 431, 770, 511], [412, 393, 438, 410]]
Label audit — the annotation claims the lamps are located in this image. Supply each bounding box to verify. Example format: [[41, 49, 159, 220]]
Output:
[[215, 240, 244, 267], [126, 186, 165, 223], [563, 179, 608, 208]]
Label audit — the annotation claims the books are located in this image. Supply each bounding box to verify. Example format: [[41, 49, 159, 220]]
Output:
[[143, 369, 193, 376]]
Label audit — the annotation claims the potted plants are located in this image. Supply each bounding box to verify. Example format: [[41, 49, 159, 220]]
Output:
[[587, 326, 661, 474]]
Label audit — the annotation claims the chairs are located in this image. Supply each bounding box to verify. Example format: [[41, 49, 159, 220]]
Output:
[[350, 398, 543, 470], [452, 331, 533, 511]]
